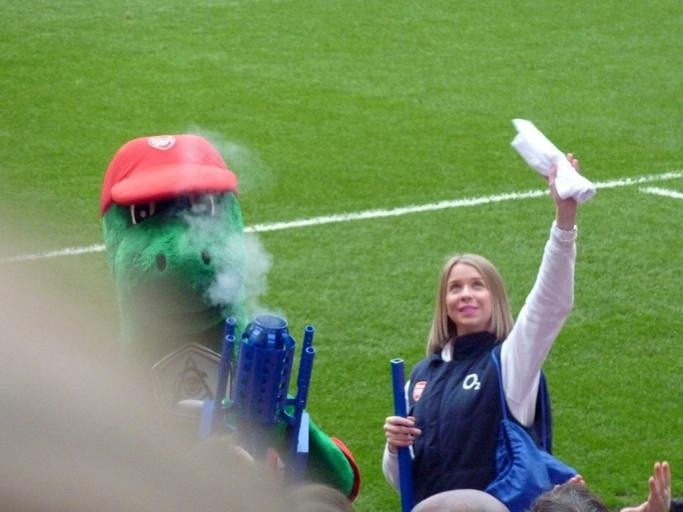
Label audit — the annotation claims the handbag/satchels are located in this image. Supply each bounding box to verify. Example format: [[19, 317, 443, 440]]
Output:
[[485, 421, 577, 512]]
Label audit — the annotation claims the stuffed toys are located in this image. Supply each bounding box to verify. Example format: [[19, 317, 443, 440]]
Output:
[[98, 133, 361, 502]]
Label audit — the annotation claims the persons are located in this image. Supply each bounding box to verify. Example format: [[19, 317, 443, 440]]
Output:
[[229, 442, 683, 512], [380, 151, 582, 509]]
